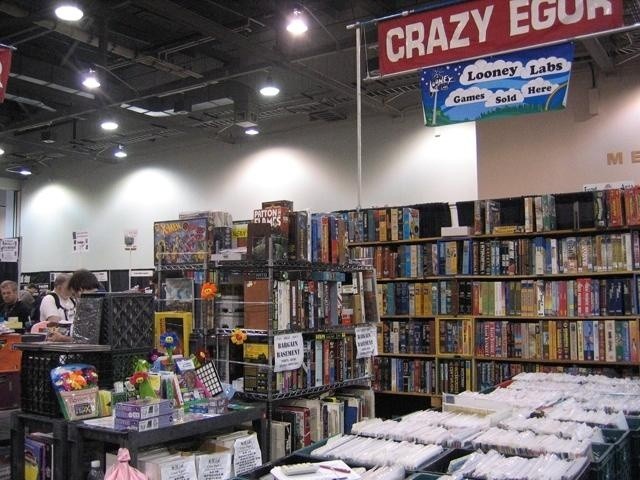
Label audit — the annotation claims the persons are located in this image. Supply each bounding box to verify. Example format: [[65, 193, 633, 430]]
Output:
[[40, 269, 106, 324], [0, 280, 30, 333], [18, 283, 37, 308]]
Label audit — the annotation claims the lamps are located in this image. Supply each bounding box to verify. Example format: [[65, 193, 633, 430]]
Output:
[[284, 6, 309, 36], [258, 76, 280, 98], [1, 146, 7, 158], [99, 113, 118, 131], [19, 164, 33, 176], [113, 144, 128, 159], [48, 0, 90, 23], [244, 126, 259, 137], [80, 65, 102, 89]]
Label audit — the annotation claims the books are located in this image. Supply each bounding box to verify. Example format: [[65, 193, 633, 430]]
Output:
[[348, 187, 640, 243], [376, 320, 639, 363], [187, 271, 218, 329], [376, 275, 638, 316], [524, 366, 639, 376], [26, 432, 55, 479], [350, 246, 639, 279], [243, 270, 380, 333], [244, 335, 370, 393], [137, 431, 263, 480], [477, 363, 524, 391], [270, 387, 375, 461], [4, 321, 23, 329], [374, 358, 470, 397], [212, 200, 349, 266]]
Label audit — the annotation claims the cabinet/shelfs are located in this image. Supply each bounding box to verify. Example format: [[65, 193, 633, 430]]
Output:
[[156, 186, 640, 462]]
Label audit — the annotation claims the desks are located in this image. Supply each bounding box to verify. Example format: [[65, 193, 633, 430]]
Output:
[[68, 401, 267, 480], [10, 410, 68, 480]]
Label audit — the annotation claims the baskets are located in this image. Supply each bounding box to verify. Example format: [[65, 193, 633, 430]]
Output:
[[230, 381, 640, 480], [228, 379, 640, 480], [21, 292, 155, 416]]
